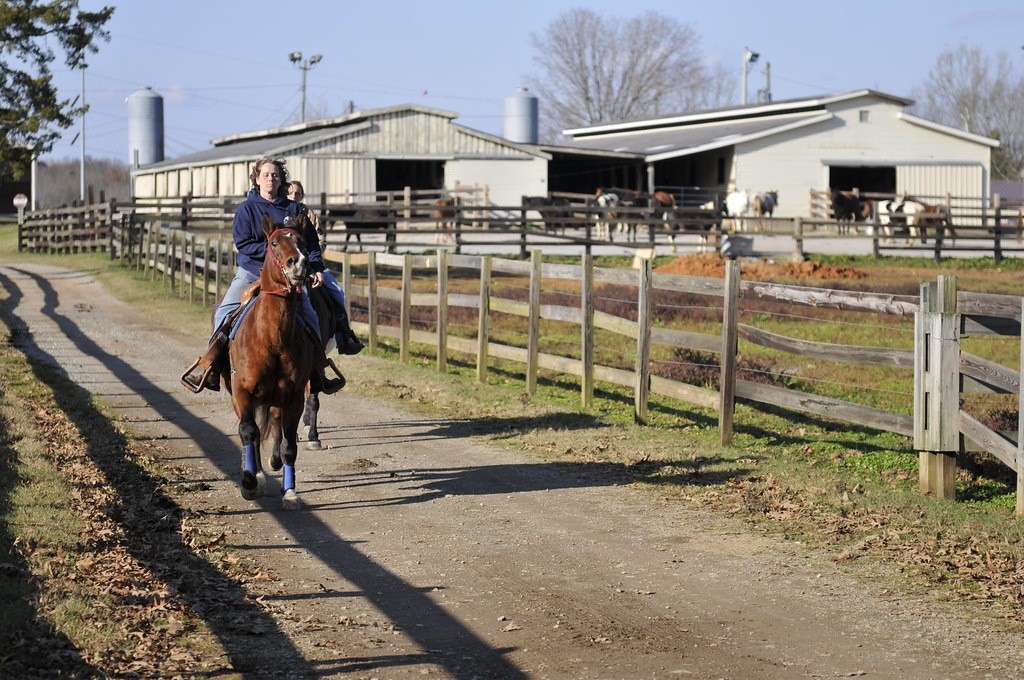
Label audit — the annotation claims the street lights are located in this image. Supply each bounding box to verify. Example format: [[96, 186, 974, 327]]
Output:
[[289, 51, 323, 122], [741, 46, 760, 106]]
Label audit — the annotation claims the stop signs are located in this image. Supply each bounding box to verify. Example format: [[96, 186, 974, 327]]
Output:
[[14, 194, 28, 209]]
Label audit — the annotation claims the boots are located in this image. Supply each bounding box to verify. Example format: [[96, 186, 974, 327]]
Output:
[[309, 373, 340, 394], [184, 361, 221, 392], [338, 313, 362, 355]]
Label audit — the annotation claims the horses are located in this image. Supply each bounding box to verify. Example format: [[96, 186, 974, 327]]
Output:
[[297, 282, 332, 450], [662, 200, 729, 253], [526, 197, 580, 236], [725, 190, 779, 234], [830, 184, 957, 248], [595, 187, 676, 243], [326, 203, 398, 254], [433, 197, 461, 244], [221, 210, 314, 512]]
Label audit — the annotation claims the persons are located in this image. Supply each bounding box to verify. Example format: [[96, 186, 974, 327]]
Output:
[[185, 158, 342, 395], [287, 181, 361, 355]]
